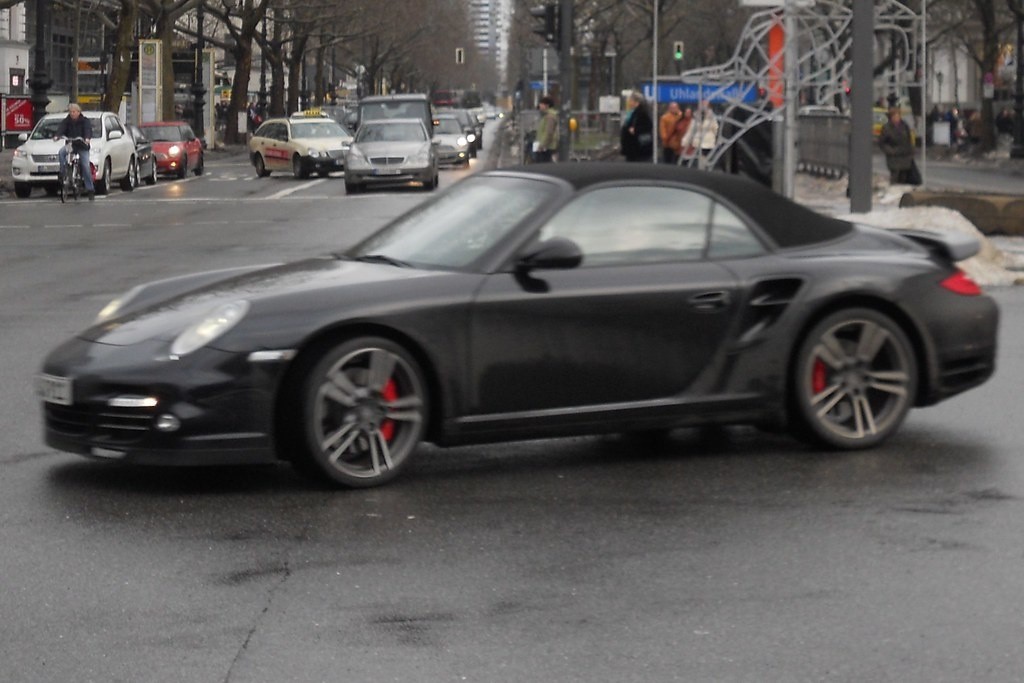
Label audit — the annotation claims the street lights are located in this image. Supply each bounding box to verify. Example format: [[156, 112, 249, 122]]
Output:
[[937, 72, 944, 112]]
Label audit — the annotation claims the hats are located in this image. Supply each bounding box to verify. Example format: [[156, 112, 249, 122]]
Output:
[[540, 97, 554, 107], [888, 107, 900, 117], [632, 92, 645, 105]]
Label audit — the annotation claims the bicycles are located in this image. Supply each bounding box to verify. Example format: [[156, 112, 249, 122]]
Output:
[[55, 135, 89, 203]]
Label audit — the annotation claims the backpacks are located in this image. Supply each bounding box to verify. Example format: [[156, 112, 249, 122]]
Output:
[[908, 160, 922, 185]]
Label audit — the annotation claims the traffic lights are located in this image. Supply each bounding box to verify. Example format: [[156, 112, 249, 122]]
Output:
[[456, 48, 463, 63], [530, 3, 560, 43], [674, 42, 683, 59]]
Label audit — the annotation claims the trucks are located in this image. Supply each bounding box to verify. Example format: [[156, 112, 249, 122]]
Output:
[[432, 90, 480, 109]]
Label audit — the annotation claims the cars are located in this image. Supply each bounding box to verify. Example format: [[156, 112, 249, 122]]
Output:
[[432, 114, 472, 167], [138, 121, 203, 179], [487, 109, 495, 120], [465, 110, 485, 150], [249, 110, 353, 179], [800, 106, 840, 115], [34, 161, 998, 490], [12, 112, 137, 198], [437, 111, 479, 158], [341, 118, 441, 194], [354, 93, 440, 139], [125, 122, 157, 187]]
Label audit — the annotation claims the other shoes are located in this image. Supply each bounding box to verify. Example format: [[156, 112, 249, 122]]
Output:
[[59, 174, 63, 184], [89, 193, 94, 200]]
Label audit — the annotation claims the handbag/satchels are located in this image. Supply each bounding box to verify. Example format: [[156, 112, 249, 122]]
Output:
[[640, 135, 653, 156]]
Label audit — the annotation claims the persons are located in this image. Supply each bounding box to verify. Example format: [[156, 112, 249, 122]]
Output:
[[931, 105, 1017, 144], [620, 95, 653, 162], [534, 97, 559, 162], [53, 104, 95, 200], [215, 101, 230, 132], [660, 99, 719, 169], [247, 102, 270, 135], [880, 109, 914, 184]]
